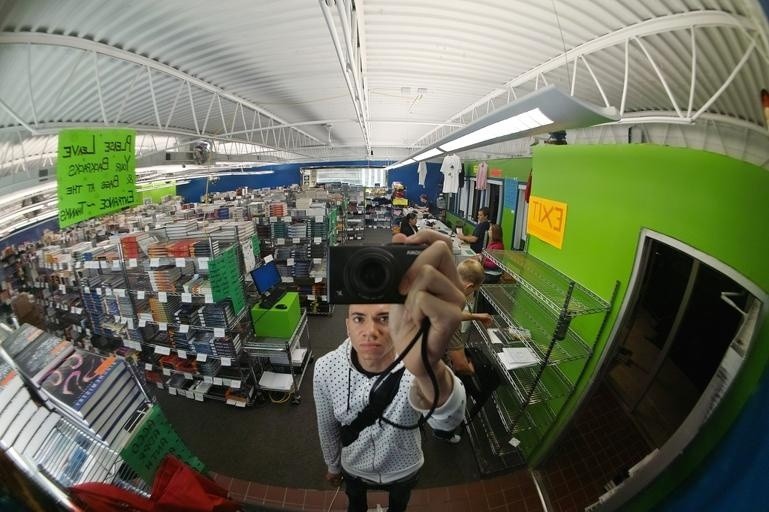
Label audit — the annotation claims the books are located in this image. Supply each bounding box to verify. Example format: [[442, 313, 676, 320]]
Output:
[[495, 352, 538, 370], [498, 326, 531, 344], [503, 348, 538, 364], [0, 184, 328, 408], [485, 326, 506, 345], [0, 322, 152, 511]]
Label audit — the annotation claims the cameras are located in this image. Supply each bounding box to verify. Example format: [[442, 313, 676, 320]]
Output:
[[327, 245, 426, 305]]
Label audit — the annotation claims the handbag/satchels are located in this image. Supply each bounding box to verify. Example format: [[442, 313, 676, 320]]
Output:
[[484, 269, 503, 284], [451, 342, 502, 406]]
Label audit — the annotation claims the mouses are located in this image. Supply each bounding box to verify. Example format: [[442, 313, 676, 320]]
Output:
[[276, 304, 287, 309]]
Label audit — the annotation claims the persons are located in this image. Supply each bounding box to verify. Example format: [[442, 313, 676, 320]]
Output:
[[415, 194, 432, 213], [477, 224, 504, 315], [456, 207, 490, 255], [399, 213, 418, 237], [432, 258, 493, 445], [310, 230, 468, 509]]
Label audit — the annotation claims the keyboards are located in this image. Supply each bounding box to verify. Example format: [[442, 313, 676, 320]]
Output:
[[259, 289, 287, 309]]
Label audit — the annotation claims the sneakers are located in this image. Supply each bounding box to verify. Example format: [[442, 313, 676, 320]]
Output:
[[431, 430, 461, 444]]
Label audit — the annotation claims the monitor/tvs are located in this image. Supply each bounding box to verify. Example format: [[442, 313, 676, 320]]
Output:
[[250, 260, 282, 302]]
